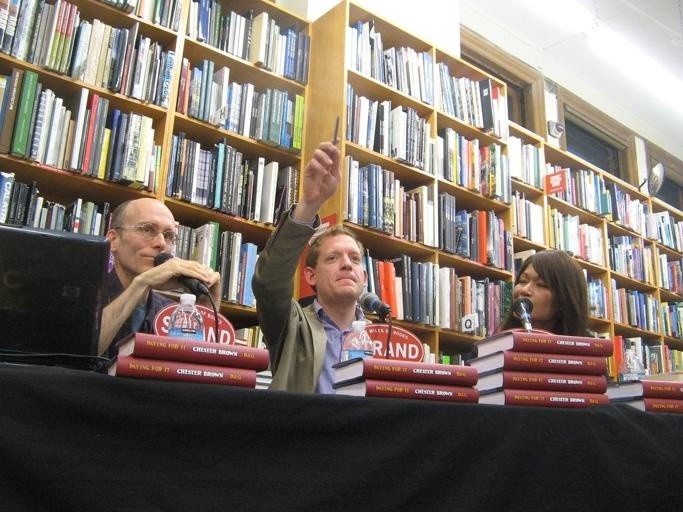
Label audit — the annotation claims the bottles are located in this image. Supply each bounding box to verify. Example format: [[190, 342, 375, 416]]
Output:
[[340, 320, 375, 361], [168, 292, 206, 342], [618, 348, 647, 382]]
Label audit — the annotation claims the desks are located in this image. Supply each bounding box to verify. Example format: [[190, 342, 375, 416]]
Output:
[[0, 358, 683, 512]]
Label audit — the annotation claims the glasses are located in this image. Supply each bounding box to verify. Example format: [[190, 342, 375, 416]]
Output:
[[116, 223, 178, 247]]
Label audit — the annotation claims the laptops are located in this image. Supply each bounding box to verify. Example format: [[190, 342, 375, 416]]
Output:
[[1, 224, 110, 369]]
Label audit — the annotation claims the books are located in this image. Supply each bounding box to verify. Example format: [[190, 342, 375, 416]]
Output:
[[1, 1, 681, 413]]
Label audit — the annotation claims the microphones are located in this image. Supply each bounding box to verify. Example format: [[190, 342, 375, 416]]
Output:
[[360, 294, 391, 319], [153, 253, 206, 298], [513, 298, 534, 331]]
